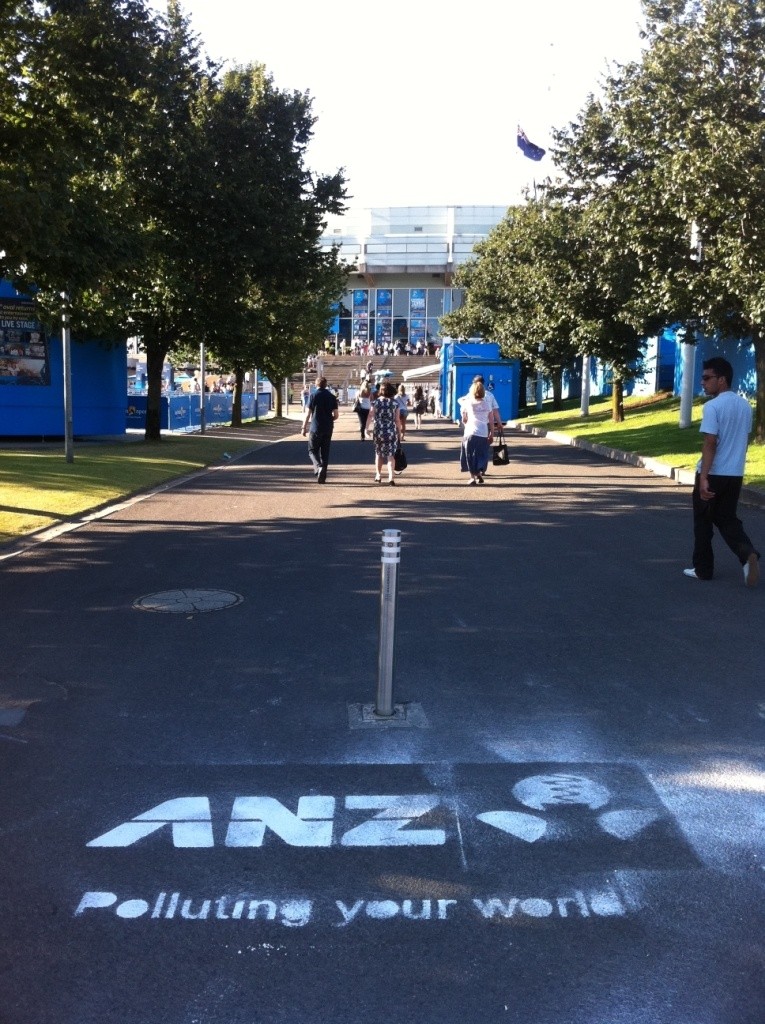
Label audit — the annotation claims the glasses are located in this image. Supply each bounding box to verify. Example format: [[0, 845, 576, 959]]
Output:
[[702, 375, 721, 381]]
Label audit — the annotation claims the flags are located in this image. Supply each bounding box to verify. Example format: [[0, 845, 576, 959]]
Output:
[[517, 126, 546, 161]]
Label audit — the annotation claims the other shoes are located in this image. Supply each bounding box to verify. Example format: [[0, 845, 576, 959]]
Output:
[[389, 481, 396, 486], [318, 467, 328, 484], [375, 478, 381, 482], [684, 568, 700, 578], [477, 475, 484, 483], [470, 481, 476, 485], [743, 553, 758, 584]]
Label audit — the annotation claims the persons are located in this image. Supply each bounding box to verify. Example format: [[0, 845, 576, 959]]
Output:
[[460, 382, 494, 483], [430, 385, 442, 419], [302, 385, 310, 413], [205, 375, 234, 394], [341, 339, 346, 356], [359, 368, 366, 384], [374, 384, 381, 398], [365, 381, 402, 486], [393, 385, 410, 441], [367, 360, 373, 377], [424, 390, 428, 416], [354, 339, 430, 356], [466, 375, 503, 475], [412, 386, 424, 429], [325, 384, 339, 398], [188, 378, 200, 392], [308, 354, 316, 368], [324, 339, 330, 354], [356, 381, 374, 441], [301, 377, 338, 484], [683, 357, 761, 588], [30, 332, 43, 343], [288, 383, 295, 404]]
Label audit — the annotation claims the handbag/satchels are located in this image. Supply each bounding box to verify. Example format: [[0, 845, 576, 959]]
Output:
[[395, 442, 408, 471], [494, 433, 509, 465], [353, 399, 360, 412]]
